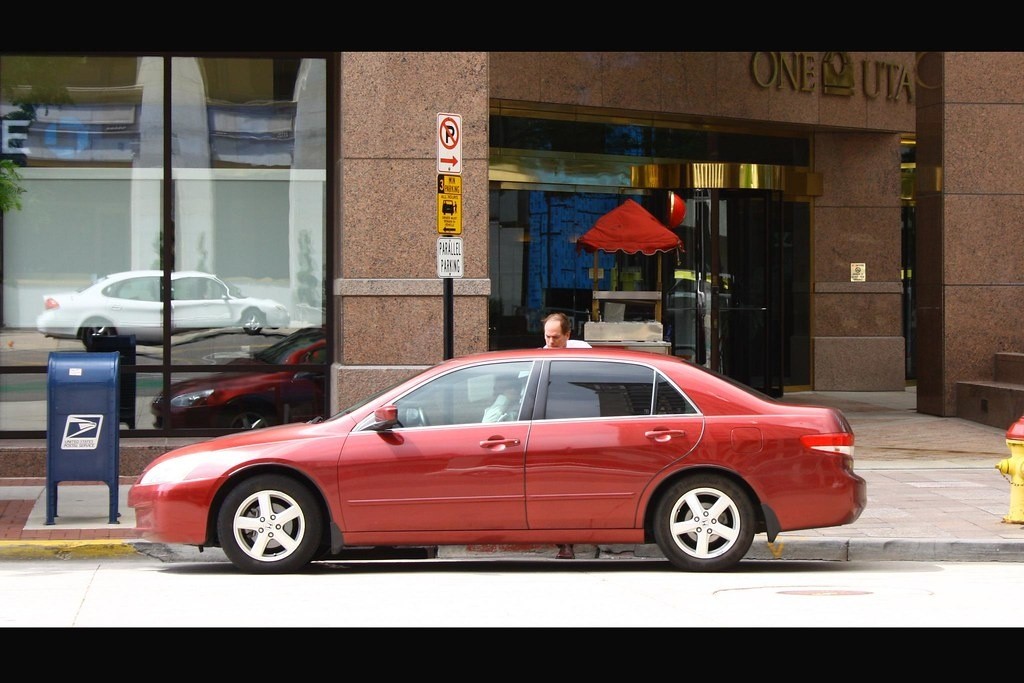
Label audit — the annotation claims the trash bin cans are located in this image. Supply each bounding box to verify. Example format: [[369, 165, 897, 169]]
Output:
[[85, 333, 137, 429], [44, 350, 121, 528]]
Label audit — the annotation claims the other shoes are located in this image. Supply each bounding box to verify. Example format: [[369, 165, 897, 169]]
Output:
[[555, 543, 575, 559]]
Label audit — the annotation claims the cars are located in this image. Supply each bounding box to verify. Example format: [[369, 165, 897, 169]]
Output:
[[126, 345, 867, 577], [36, 270, 294, 353], [152, 327, 328, 430]]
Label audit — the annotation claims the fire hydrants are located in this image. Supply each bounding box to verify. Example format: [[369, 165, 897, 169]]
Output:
[[993, 416, 1024, 526]]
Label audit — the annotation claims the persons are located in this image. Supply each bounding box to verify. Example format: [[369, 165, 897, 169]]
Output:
[[540, 312, 602, 559], [481, 370, 522, 423]]
